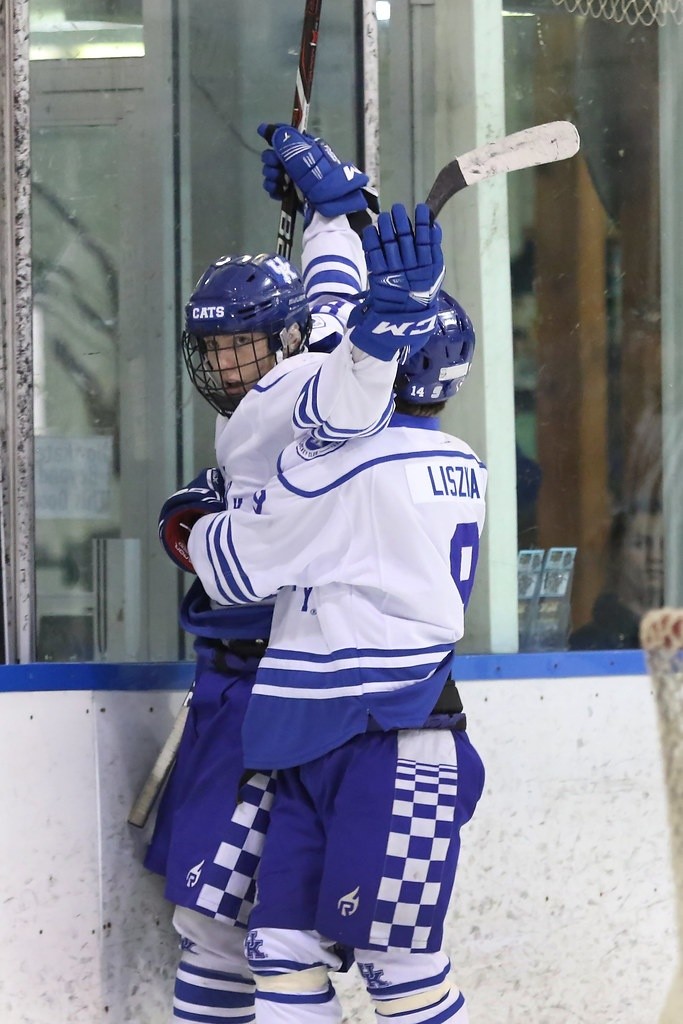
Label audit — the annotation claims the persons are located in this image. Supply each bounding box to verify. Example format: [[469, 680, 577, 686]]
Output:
[[127, 122, 444, 1024], [567, 500, 663, 651], [159, 285, 488, 1024]]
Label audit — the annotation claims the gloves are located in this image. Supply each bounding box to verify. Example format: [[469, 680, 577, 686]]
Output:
[[345, 203, 447, 364], [258, 123, 381, 233], [159, 467, 225, 575]]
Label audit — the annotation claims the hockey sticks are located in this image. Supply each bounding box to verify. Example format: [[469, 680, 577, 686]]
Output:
[[421, 117, 583, 225], [127, 1, 322, 829]]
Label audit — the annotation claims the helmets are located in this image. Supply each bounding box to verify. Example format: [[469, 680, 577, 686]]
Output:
[[393, 288, 476, 404], [180, 252, 308, 418]]
